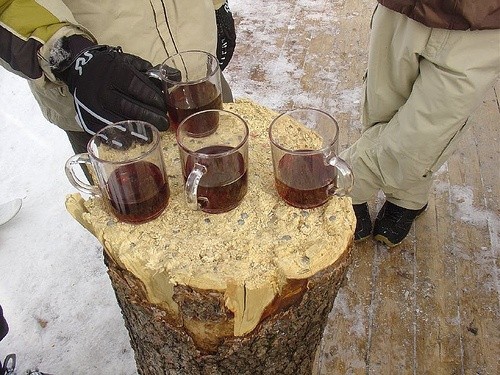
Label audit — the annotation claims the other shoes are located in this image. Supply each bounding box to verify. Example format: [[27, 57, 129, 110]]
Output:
[[373, 200, 429, 248], [352, 202, 373, 243]]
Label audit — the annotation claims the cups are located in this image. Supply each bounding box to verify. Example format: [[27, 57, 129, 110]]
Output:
[[270, 107, 355, 209], [176, 108, 249, 213], [145, 49, 223, 138], [64, 120, 170, 223]]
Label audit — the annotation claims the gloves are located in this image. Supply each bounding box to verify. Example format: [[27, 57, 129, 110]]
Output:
[[215, 0, 236, 71], [50, 34, 182, 150]]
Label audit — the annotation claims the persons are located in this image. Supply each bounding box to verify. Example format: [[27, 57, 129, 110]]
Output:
[[338, 0, 500, 248], [0, 0, 236, 187]]
